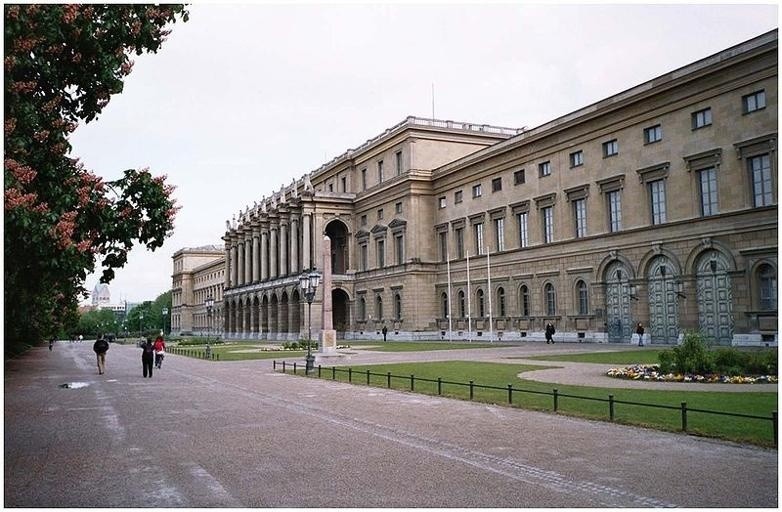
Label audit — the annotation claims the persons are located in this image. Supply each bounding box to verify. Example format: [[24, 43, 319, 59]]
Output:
[[94, 332, 109, 375], [546, 322, 555, 344], [70, 333, 84, 342], [636, 322, 645, 347], [140, 337, 155, 378], [382, 325, 387, 342], [154, 335, 166, 370], [49, 334, 58, 351]]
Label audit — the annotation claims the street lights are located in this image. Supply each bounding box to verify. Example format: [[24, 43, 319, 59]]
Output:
[[203, 287, 215, 351], [137, 309, 146, 346], [160, 302, 168, 355], [94, 315, 132, 344], [299, 262, 322, 376]]
